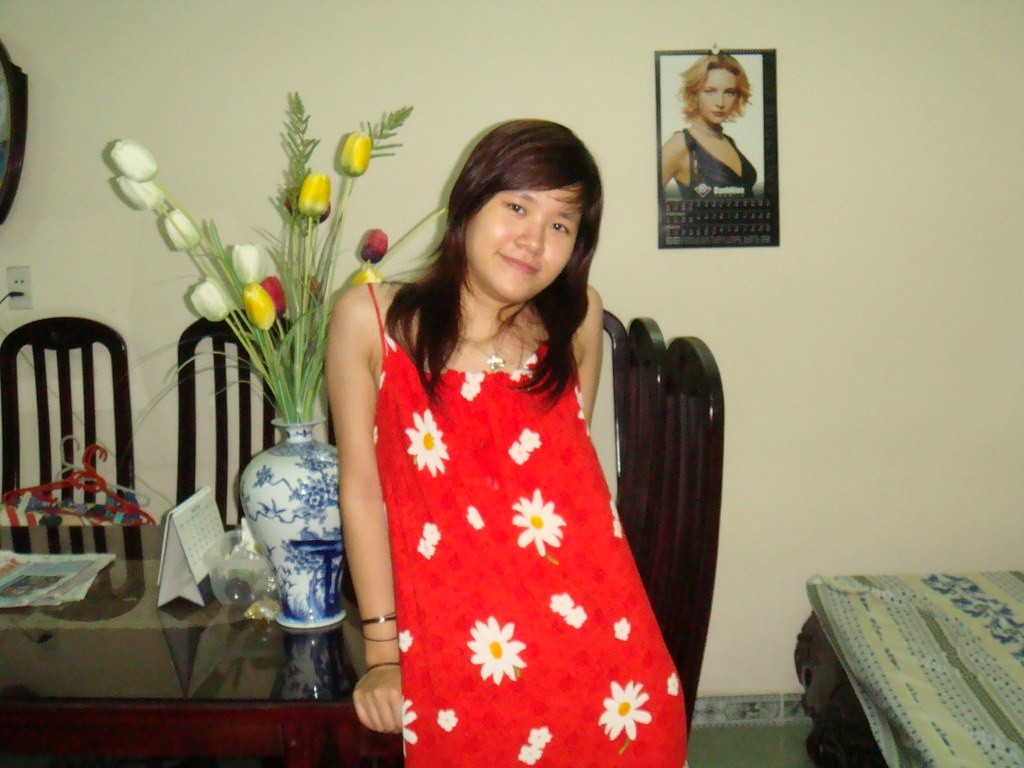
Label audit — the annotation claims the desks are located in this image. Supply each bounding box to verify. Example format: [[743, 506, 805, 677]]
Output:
[[0, 524, 401, 768], [793, 565, 1024, 768]]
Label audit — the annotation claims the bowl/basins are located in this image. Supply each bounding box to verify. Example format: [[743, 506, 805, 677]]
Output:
[[204, 529, 282, 606]]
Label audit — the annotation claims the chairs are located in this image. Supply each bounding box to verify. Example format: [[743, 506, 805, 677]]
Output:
[[13, 525, 146, 623], [601, 307, 725, 746], [176, 311, 337, 533], [0, 317, 139, 524]]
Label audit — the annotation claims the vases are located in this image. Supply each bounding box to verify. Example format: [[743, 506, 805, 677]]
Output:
[[239, 418, 348, 629]]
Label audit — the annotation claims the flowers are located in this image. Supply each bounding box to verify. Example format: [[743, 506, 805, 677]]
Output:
[[108, 91, 449, 423]]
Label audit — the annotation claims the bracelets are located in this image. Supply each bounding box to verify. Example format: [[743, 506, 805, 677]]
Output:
[[361, 612, 396, 626], [364, 637, 397, 642]]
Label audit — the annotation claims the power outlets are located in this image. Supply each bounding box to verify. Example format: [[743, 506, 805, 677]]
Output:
[[8, 267, 34, 310]]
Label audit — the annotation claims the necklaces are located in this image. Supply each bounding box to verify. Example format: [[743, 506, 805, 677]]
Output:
[[458, 327, 508, 372]]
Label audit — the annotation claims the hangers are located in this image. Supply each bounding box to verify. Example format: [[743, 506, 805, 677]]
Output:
[[2, 435, 157, 525]]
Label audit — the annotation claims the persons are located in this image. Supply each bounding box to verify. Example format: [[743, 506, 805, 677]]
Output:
[[325, 119, 688, 768], [662, 53, 757, 200]]
